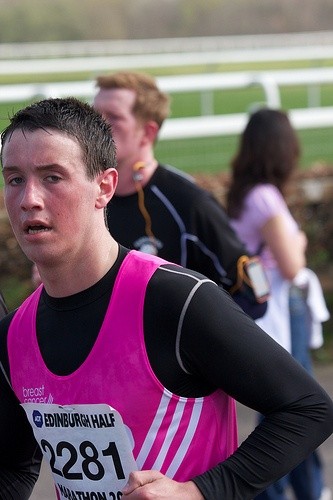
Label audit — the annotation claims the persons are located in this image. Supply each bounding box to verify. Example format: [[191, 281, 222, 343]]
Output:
[[89, 72, 269, 321], [0, 98, 333, 500], [226, 109, 324, 500]]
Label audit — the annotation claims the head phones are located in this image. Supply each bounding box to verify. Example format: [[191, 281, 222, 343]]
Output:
[[133, 162, 144, 172]]
